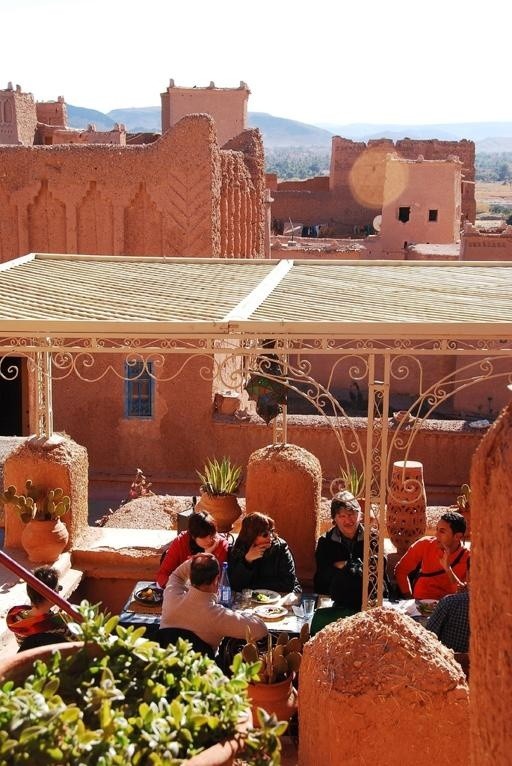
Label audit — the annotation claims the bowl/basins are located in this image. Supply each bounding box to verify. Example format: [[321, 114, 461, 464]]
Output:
[[134, 587, 163, 607], [416, 604, 438, 616]]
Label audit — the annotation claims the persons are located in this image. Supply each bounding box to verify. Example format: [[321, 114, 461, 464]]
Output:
[[313, 490, 389, 598], [154, 509, 230, 589], [394, 512, 471, 599], [227, 511, 302, 594], [426, 557, 471, 653], [6, 563, 74, 646], [159, 552, 269, 657]]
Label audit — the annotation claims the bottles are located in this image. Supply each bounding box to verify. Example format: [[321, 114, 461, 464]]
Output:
[[218, 561, 232, 606]]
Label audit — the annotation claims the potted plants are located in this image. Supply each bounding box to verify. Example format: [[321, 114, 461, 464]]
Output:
[[1, 597, 294, 765], [235, 621, 312, 729], [455, 482, 471, 539], [338, 463, 369, 514], [192, 455, 245, 534], [2, 476, 77, 567]]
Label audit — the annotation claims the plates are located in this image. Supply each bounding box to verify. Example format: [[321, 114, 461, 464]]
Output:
[[254, 604, 288, 618], [251, 588, 281, 603]]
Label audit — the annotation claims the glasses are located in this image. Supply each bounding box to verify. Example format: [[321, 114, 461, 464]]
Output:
[[258, 529, 275, 539]]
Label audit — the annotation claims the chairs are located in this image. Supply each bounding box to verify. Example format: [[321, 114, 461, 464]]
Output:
[[157, 627, 212, 659], [16, 634, 67, 654], [158, 541, 421, 599], [452, 652, 471, 685]]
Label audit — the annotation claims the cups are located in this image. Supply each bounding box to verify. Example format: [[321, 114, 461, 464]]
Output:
[[304, 598, 332, 620], [242, 588, 253, 608]]
